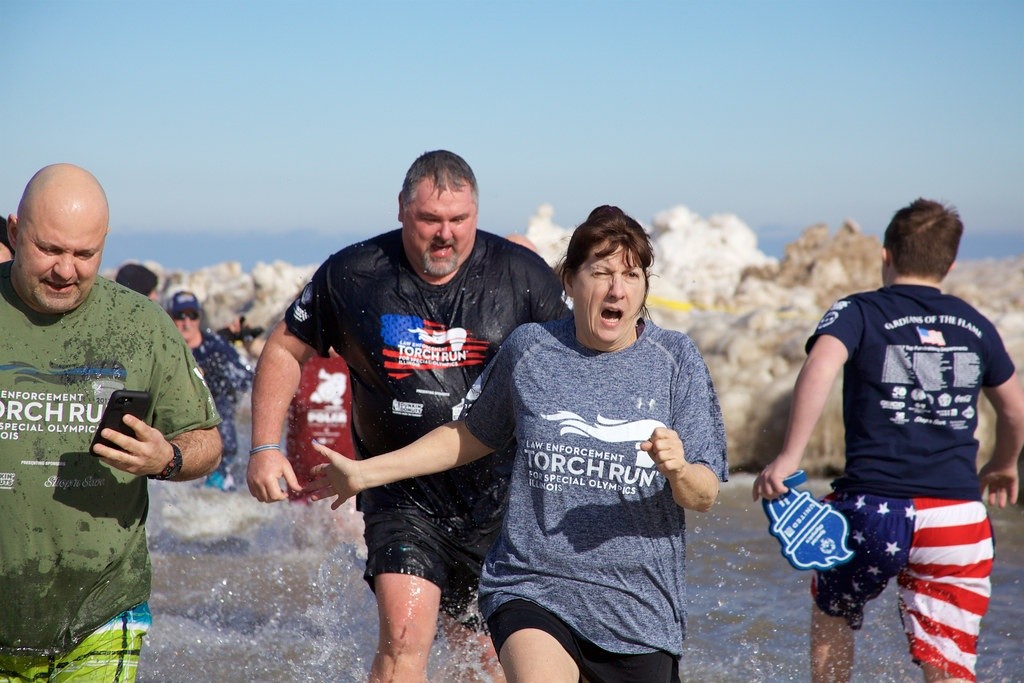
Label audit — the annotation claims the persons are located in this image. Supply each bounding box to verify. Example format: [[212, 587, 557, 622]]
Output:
[[300, 204, 729, 683], [0, 163, 224, 683], [116, 264, 160, 301], [750, 200, 1024, 683], [168, 290, 253, 493], [245, 152, 571, 682], [0, 216, 15, 266]]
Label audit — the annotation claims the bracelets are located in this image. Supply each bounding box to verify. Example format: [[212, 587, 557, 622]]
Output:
[[249, 444, 279, 455]]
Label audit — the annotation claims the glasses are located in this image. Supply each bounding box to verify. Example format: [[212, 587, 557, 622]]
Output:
[[173, 311, 199, 320]]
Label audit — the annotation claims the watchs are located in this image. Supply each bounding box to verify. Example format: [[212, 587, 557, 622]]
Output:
[[148, 443, 184, 481]]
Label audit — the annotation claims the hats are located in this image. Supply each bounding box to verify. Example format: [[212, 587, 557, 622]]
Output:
[[169, 293, 202, 318], [116, 264, 157, 298]]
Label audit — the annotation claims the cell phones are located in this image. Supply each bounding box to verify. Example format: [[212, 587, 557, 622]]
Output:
[[88, 390, 152, 458]]
